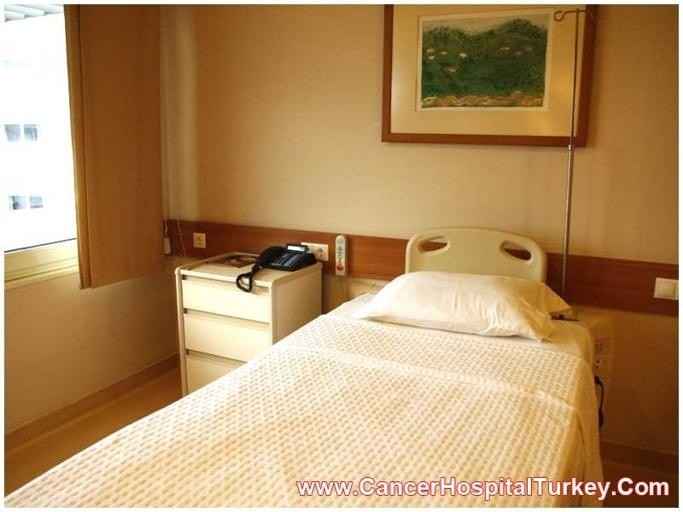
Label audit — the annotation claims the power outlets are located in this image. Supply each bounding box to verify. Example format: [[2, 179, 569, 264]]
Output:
[[192, 232, 206, 249], [590, 337, 609, 414], [300, 242, 329, 262]]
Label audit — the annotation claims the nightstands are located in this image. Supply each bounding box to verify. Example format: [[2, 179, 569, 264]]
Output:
[[173, 252, 323, 399]]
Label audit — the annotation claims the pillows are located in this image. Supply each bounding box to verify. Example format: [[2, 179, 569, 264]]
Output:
[[352, 270, 575, 343]]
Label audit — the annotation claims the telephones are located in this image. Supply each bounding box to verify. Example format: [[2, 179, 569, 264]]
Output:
[[256, 244, 315, 271]]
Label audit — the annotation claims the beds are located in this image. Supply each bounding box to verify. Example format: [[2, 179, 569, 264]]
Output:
[[4, 227, 606, 508]]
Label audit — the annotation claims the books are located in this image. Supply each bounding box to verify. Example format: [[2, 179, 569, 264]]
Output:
[[207, 255, 259, 270]]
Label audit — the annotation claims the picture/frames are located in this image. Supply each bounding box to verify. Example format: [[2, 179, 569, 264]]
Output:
[[381, 5, 598, 147]]
